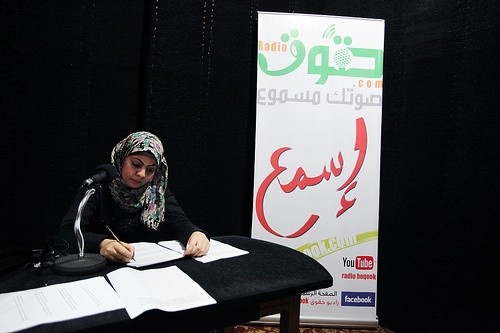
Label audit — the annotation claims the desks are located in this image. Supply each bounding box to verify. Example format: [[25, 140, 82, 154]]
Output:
[[1, 235, 334, 333]]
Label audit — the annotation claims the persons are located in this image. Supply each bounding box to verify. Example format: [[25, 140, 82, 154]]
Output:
[[58, 131, 211, 263]]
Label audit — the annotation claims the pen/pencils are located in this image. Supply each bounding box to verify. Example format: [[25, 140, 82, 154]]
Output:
[[105, 225, 136, 261]]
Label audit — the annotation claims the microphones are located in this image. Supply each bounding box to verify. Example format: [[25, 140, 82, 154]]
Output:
[[84, 163, 117, 187]]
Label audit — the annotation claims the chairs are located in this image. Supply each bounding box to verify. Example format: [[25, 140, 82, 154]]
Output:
[[32, 175, 86, 249]]
